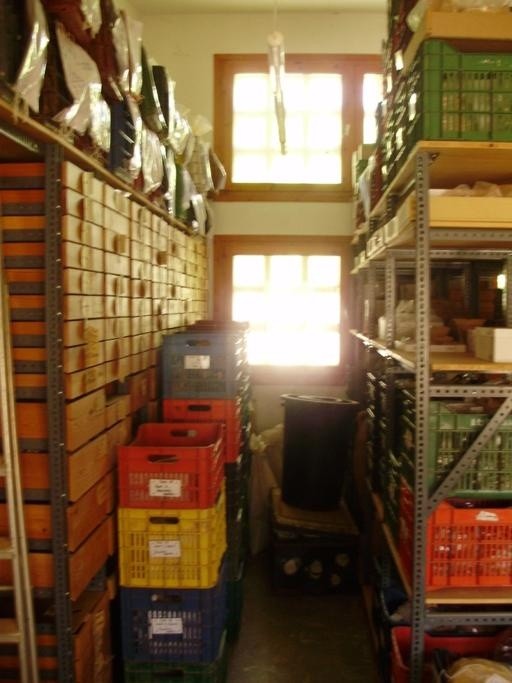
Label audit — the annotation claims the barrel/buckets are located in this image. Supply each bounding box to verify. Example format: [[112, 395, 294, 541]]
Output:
[[280, 393, 360, 510]]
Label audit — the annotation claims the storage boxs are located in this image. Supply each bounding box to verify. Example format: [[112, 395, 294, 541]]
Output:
[[269, 487, 354, 584]]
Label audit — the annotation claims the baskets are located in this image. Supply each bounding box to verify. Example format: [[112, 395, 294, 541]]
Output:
[[383, 40, 511, 191], [367, 369, 512, 590], [389, 624, 512, 683], [116, 327, 251, 683], [116, 327, 251, 676]]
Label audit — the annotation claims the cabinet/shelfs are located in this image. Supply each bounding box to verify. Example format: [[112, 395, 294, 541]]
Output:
[[0, 100, 212, 683], [348, 10, 512, 683]]
